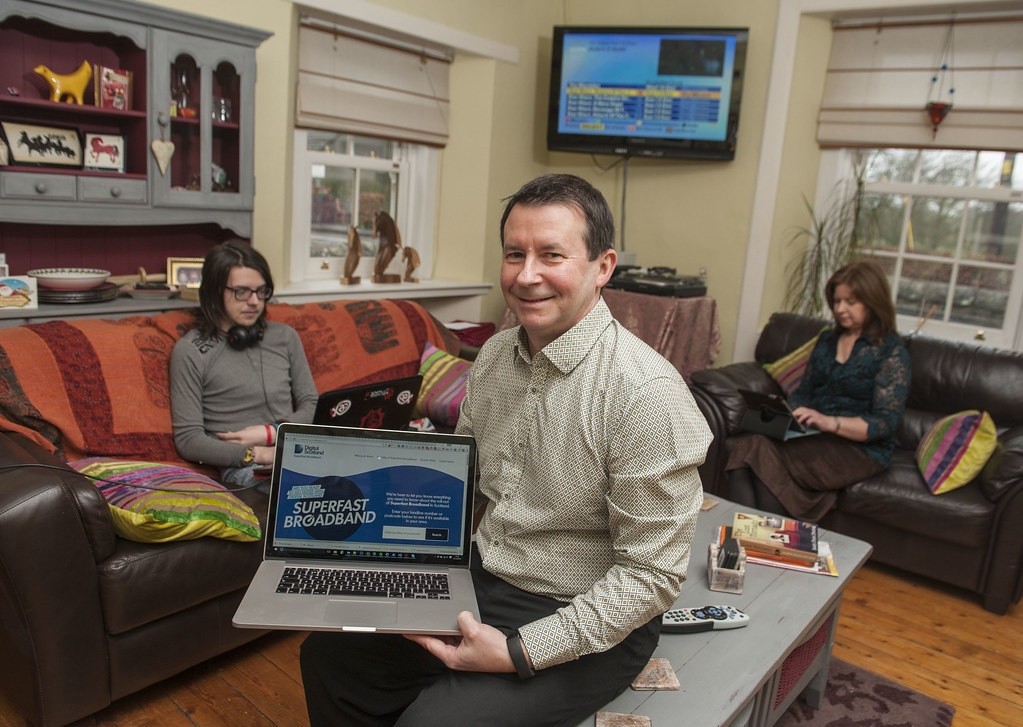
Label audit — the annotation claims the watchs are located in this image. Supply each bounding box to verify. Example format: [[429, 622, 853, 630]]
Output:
[[242, 445, 256, 466]]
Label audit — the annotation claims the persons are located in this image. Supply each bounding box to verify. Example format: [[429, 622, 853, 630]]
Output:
[[168, 240, 320, 494], [722, 253, 910, 521], [297, 175, 713, 727]]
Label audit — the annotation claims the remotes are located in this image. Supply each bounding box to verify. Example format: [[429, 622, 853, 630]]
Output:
[[660, 605, 750, 633]]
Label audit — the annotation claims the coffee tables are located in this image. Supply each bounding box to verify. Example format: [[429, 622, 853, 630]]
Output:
[[574, 491, 873, 727]]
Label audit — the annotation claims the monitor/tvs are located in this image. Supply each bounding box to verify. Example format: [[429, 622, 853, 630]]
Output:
[[549, 26, 750, 160]]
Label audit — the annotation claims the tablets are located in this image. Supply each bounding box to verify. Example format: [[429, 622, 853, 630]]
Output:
[[738, 388, 806, 434]]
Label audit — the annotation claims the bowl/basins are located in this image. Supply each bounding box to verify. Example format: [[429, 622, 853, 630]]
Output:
[[27, 268, 111, 291]]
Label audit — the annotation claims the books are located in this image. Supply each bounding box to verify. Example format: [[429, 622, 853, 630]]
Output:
[[718, 509, 839, 578]]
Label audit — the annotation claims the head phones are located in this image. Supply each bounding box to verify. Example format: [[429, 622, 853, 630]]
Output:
[[213, 318, 264, 350]]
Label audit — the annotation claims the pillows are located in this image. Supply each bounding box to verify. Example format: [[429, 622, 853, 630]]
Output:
[[763, 325, 830, 398], [64, 457, 262, 545], [414, 343, 474, 426], [913, 409, 998, 495]]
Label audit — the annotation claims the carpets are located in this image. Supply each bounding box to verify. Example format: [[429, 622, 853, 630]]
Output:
[[775, 654, 957, 727]]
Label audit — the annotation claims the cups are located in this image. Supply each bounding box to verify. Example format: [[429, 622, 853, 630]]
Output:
[[214, 97, 233, 123]]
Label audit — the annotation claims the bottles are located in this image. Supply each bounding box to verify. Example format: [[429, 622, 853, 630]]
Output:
[[173, 57, 191, 113]]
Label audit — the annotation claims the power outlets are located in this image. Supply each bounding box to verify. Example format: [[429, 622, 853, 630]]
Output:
[[698, 267, 708, 287]]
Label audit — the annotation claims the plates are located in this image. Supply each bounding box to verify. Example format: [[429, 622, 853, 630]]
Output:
[[37, 283, 122, 304], [120, 282, 186, 300]]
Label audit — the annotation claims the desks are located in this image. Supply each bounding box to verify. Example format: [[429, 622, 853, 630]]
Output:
[[494, 288, 720, 385]]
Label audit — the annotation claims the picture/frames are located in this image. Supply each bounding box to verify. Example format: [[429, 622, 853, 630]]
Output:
[[0, 277, 38, 310], [0, 116, 85, 170], [167, 256, 206, 289], [83, 129, 126, 173]]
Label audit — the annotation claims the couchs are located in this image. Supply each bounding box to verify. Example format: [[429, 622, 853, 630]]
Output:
[[0, 298, 480, 727], [689, 311, 1023, 617]]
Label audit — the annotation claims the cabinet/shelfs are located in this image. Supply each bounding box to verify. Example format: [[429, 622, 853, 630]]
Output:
[[0, 0, 275, 275]]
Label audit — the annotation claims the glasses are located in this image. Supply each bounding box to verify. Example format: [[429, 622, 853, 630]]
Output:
[[225, 286, 273, 301]]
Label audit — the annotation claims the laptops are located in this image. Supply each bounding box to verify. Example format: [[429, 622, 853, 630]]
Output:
[[232, 423, 482, 635], [253, 375, 425, 474]]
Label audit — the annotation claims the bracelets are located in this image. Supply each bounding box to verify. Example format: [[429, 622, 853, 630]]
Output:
[[835, 416, 840, 433], [265, 424, 272, 447], [506, 632, 534, 680], [270, 421, 279, 433]]
